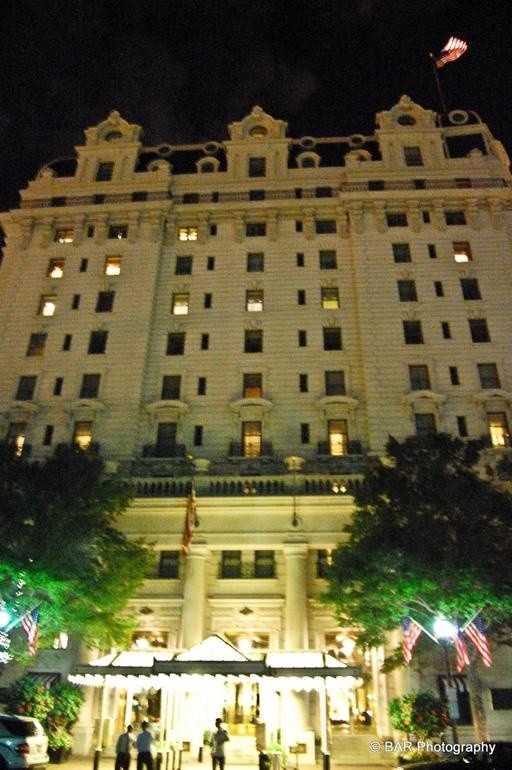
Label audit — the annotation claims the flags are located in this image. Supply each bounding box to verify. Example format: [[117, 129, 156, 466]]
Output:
[[464, 612, 495, 667], [180, 478, 195, 558], [450, 618, 472, 674], [401, 615, 422, 666], [22, 605, 40, 655], [431, 36, 467, 68]]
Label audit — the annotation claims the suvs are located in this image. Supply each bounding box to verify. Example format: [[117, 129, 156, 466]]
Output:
[[0, 714, 49, 770]]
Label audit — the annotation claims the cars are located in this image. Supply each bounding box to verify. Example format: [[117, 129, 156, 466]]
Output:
[[392, 742, 511, 769]]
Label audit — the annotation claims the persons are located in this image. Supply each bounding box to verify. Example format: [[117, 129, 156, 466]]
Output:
[[134, 720, 154, 770], [114, 725, 134, 770], [209, 718, 230, 770]]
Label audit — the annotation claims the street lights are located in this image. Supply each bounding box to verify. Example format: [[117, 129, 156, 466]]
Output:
[[434, 618, 460, 748]]
